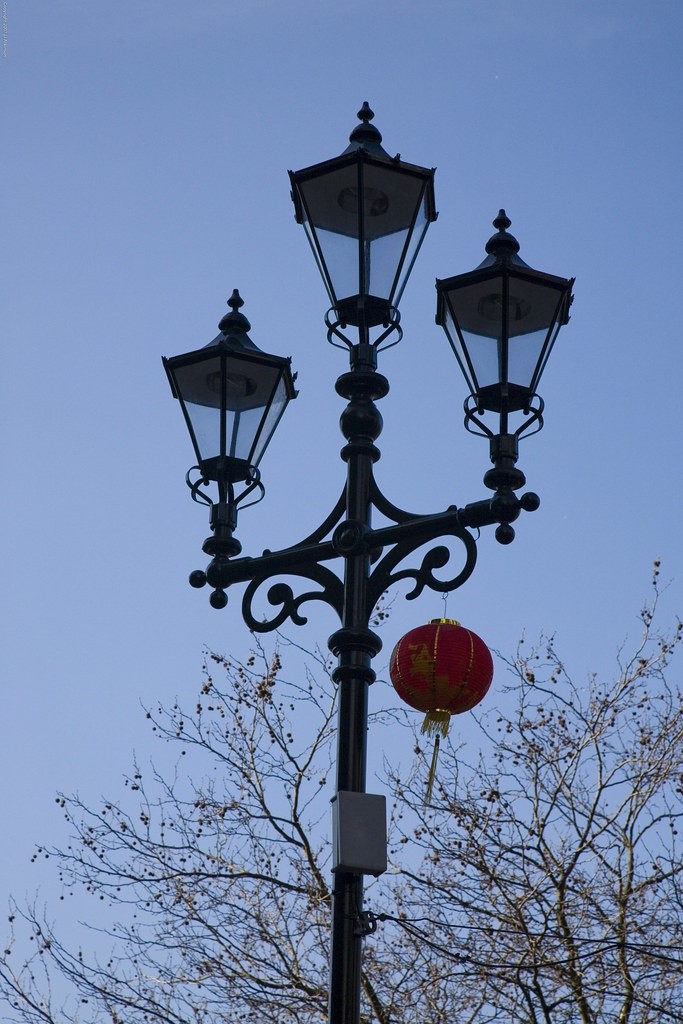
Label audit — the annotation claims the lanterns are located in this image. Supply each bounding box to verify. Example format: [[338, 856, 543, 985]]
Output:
[[389, 617, 494, 806]]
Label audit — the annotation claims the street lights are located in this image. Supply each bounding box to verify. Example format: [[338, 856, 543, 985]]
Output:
[[161, 96, 580, 1023]]
